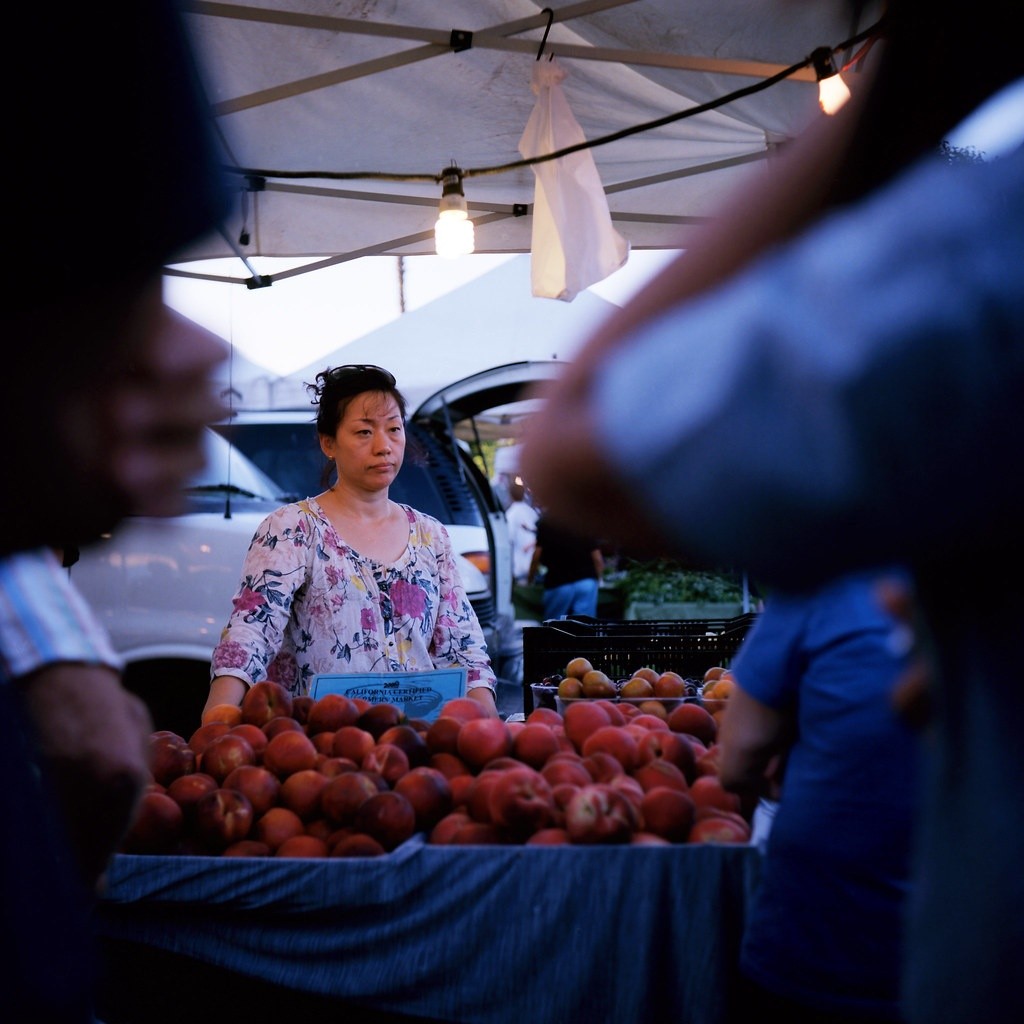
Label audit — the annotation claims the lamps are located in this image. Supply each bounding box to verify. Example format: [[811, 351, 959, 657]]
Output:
[[433, 165, 479, 274], [810, 44, 851, 118]]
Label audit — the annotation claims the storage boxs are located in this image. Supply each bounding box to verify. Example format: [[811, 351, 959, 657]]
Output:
[[75, 655, 776, 1023]]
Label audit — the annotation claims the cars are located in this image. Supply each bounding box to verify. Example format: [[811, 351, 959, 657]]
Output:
[[66, 357, 574, 746]]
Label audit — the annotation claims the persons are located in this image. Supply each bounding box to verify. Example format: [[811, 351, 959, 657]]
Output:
[[0, 0, 251, 574], [714, 559, 938, 1024], [505, 0, 1024, 1024], [505, 480, 543, 587], [0, 544, 149, 911], [526, 504, 606, 622], [200, 363, 502, 722]]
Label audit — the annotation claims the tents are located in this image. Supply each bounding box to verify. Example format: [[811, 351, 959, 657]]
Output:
[[214, 241, 625, 436], [158, 0, 862, 292]]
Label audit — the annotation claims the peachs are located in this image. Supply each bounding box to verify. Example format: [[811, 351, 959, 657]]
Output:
[[131, 680, 749, 858]]
